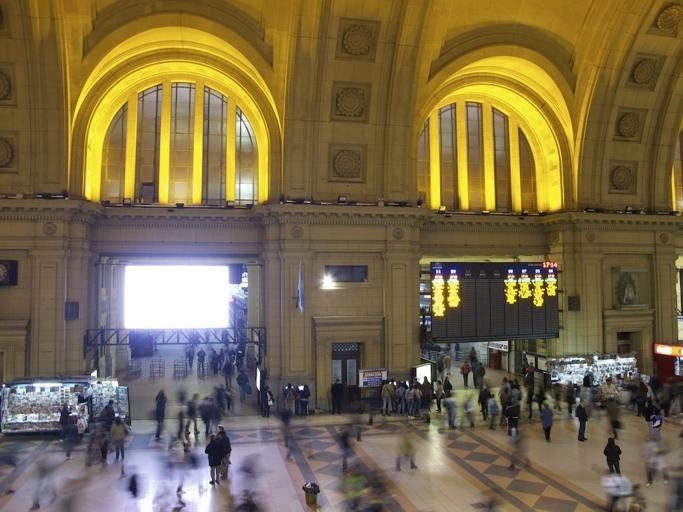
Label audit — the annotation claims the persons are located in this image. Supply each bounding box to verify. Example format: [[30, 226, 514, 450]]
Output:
[[1, 344, 682, 512]]
